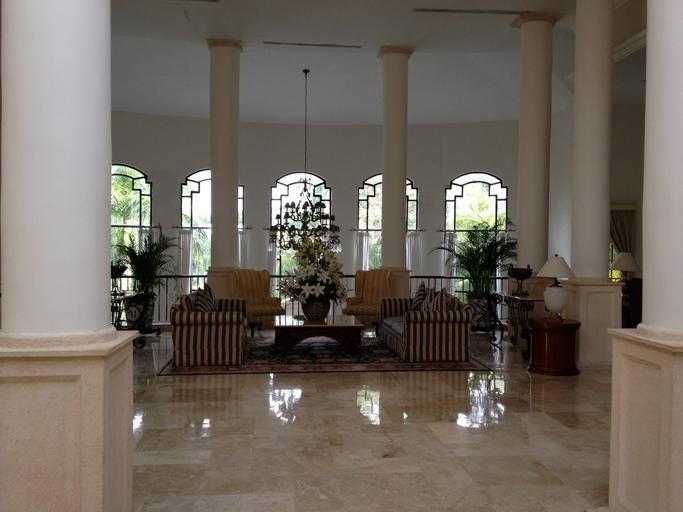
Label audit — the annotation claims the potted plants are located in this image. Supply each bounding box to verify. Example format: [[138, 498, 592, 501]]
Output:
[[112, 224, 185, 333], [429, 220, 519, 333]]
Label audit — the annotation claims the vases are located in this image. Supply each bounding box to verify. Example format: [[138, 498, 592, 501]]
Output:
[[297, 298, 332, 322]]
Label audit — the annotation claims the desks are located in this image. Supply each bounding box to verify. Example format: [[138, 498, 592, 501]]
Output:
[[109, 288, 162, 348], [483, 287, 544, 346], [269, 313, 365, 356]]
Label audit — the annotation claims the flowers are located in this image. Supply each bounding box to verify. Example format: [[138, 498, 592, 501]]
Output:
[[277, 238, 352, 304]]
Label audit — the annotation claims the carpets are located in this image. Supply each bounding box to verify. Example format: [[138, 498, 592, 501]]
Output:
[[154, 330, 494, 376]]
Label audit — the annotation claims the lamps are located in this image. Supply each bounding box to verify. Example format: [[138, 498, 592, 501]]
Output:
[[536, 252, 577, 322], [268, 69, 343, 252], [610, 250, 642, 292]]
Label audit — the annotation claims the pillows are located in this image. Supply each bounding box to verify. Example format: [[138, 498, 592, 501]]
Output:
[[410, 283, 425, 312], [430, 288, 447, 313], [194, 286, 211, 313], [178, 291, 196, 312], [420, 289, 434, 314], [204, 290, 218, 310], [446, 292, 459, 313], [203, 282, 217, 300]]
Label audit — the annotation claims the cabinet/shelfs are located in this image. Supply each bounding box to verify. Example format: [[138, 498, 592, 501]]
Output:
[[526, 317, 581, 376]]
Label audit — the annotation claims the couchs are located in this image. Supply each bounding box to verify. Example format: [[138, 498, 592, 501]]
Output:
[[379, 281, 477, 363], [342, 268, 392, 335], [229, 267, 286, 337], [168, 284, 247, 368]]
